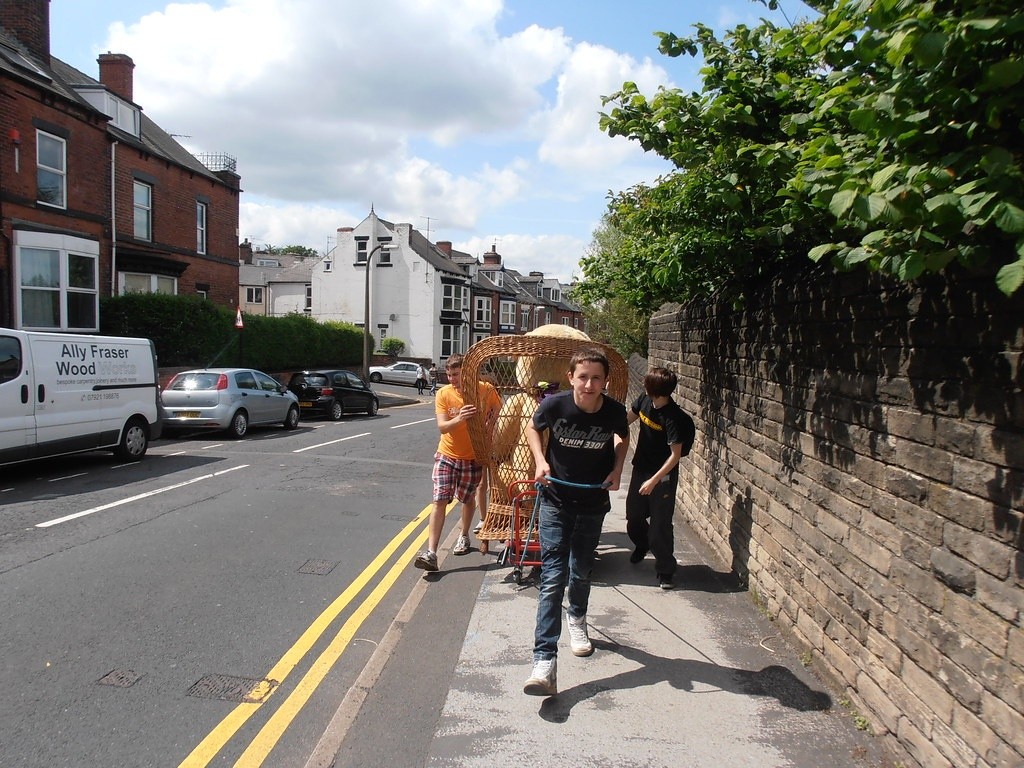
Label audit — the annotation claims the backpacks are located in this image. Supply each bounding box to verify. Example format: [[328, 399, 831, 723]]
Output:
[[638, 392, 695, 457]]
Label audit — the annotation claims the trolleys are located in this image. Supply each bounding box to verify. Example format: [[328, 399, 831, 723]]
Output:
[[497, 480, 570, 584]]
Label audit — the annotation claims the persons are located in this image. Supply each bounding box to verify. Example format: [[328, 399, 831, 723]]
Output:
[[413, 353, 487, 571], [625, 365, 684, 589], [473, 381, 502, 534], [428, 362, 438, 395], [521, 348, 630, 695], [416, 363, 427, 395]]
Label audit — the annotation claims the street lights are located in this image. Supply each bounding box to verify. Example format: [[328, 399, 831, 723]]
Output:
[[363, 244, 400, 386], [527, 306, 546, 332]]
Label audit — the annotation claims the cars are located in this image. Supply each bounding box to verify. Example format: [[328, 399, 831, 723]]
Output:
[[288, 370, 379, 421], [369, 361, 432, 388], [161, 368, 300, 440]]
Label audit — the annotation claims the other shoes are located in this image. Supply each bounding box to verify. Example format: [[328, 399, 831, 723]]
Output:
[[429, 392, 432, 396], [418, 390, 420, 395], [630, 546, 649, 563], [433, 392, 435, 395], [473, 519, 486, 534], [421, 391, 423, 395], [659, 573, 673, 588]]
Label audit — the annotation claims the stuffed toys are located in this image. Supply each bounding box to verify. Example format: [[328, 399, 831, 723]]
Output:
[[490, 323, 593, 504]]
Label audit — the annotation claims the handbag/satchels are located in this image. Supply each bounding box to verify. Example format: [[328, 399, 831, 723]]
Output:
[[421, 373, 426, 379]]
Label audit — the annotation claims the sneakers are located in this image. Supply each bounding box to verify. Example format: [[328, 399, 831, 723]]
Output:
[[524, 658, 557, 695], [414, 550, 438, 571], [566, 613, 593, 656], [453, 535, 471, 554]]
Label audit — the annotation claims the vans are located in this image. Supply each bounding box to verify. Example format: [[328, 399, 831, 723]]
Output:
[[0, 327, 162, 467]]
